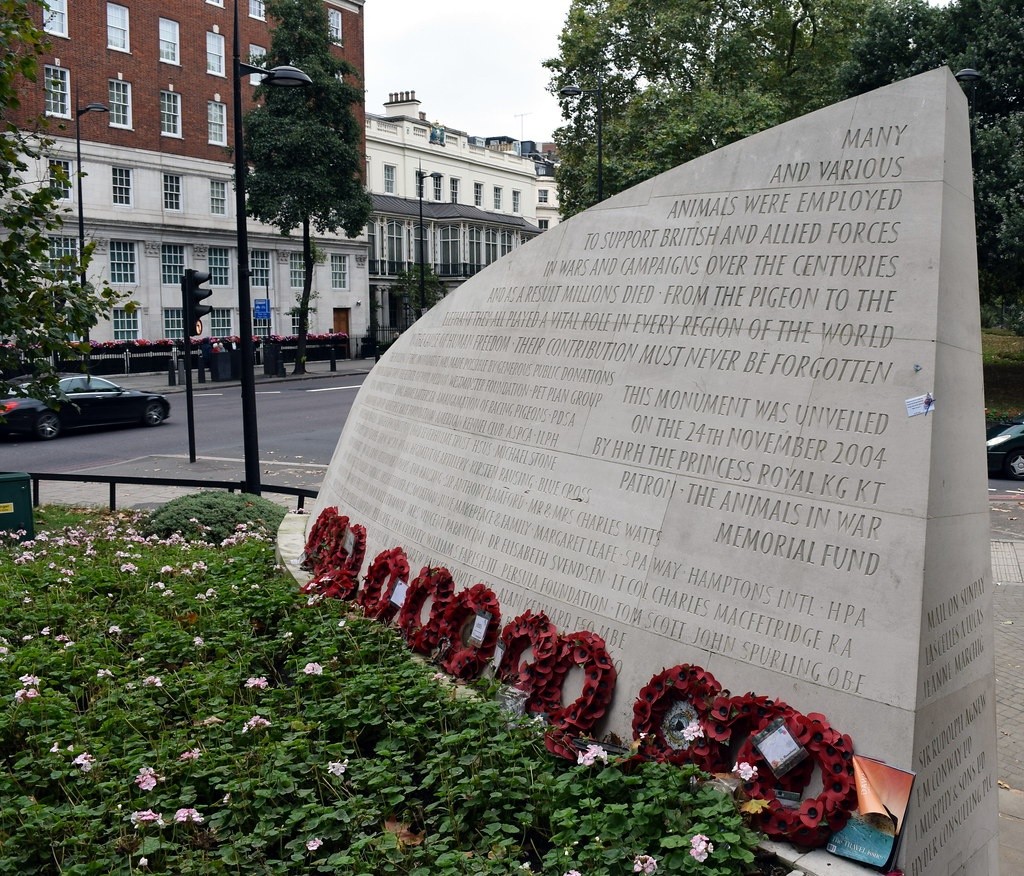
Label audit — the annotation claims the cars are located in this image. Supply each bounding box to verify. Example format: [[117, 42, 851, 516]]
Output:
[[985, 412, 1024, 481], [0, 372, 170, 439]]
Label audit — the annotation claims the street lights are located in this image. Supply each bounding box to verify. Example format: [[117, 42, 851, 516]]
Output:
[[953, 61, 984, 155], [74, 72, 110, 371], [417, 154, 443, 319], [560, 83, 604, 203], [230, 1, 314, 495]]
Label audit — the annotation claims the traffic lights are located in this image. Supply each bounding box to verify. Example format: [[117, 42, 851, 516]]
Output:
[[187, 269, 213, 322]]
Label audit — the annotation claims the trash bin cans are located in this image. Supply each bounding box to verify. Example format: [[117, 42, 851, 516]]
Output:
[[228, 349, 242, 381], [210, 351, 233, 382]]
